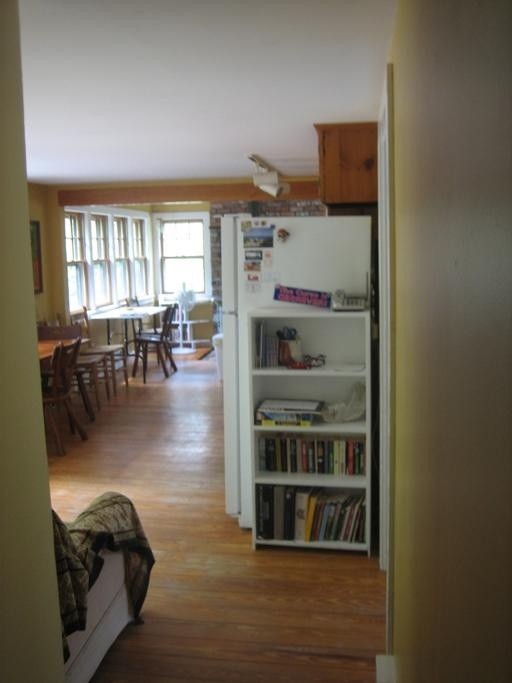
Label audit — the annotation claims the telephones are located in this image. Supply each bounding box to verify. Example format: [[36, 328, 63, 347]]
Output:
[[332, 289, 366, 311]]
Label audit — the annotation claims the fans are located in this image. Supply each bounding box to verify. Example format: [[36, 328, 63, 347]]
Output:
[[168, 288, 198, 355]]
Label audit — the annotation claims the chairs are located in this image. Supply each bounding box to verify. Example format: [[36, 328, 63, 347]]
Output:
[[35, 297, 178, 457]]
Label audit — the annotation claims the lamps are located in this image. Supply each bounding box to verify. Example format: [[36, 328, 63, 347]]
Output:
[[251, 161, 285, 199]]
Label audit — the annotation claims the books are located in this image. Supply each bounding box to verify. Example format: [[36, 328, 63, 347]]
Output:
[[257, 433, 366, 545], [255, 321, 279, 369]]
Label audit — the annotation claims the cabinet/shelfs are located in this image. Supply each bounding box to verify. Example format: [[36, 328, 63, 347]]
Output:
[[246, 307, 373, 558], [312, 121, 377, 209]]
